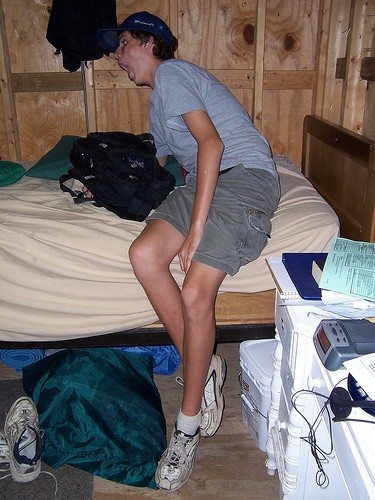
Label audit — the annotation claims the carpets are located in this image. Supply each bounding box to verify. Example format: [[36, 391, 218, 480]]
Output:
[[0, 379, 94, 500]]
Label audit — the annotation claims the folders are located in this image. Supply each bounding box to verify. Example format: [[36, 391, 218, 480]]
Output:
[[282, 252, 328, 300]]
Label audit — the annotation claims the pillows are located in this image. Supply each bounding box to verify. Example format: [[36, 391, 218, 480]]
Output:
[[21, 348, 168, 490], [24, 135, 186, 186]]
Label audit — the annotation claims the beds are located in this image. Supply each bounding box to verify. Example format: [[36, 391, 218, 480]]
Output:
[[0, 114, 375, 349]]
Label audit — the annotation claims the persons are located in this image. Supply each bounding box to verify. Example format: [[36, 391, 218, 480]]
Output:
[[82, 10, 282, 490]]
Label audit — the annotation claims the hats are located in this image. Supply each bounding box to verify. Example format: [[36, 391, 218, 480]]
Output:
[[97, 11, 176, 52]]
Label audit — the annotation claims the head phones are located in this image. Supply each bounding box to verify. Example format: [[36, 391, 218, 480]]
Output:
[[330, 387, 375, 418]]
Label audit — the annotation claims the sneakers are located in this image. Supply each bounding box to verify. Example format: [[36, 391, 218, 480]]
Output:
[[3, 396, 45, 483], [155, 420, 202, 492], [175, 355, 226, 438]]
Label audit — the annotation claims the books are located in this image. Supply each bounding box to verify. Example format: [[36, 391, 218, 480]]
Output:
[[265, 253, 330, 300]]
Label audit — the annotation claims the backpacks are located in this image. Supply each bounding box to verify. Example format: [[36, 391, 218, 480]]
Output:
[[59, 132, 176, 222]]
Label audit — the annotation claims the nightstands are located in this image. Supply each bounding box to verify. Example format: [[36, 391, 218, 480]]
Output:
[[264, 289, 375, 500]]
